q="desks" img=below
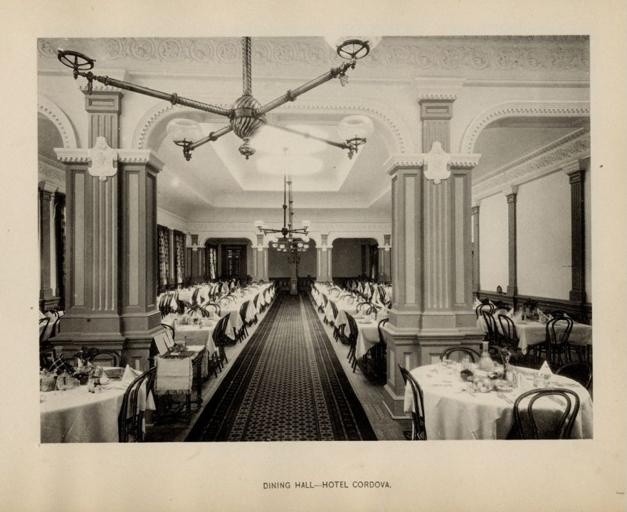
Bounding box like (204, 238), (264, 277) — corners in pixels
(407, 362), (593, 439)
(482, 307), (592, 367)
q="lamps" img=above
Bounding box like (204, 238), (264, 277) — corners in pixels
(255, 220), (310, 252)
(57, 20), (371, 160)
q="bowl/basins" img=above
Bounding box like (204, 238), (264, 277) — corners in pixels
(72, 373), (89, 386)
(106, 368), (126, 380)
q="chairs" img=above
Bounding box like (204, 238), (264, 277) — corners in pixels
(514, 387), (580, 438)
(475, 303), (526, 362)
(397, 362), (427, 440)
(309, 274), (395, 382)
(40, 313), (157, 442)
(157, 277), (275, 368)
(555, 361), (592, 389)
(440, 346), (484, 363)
(532, 316), (574, 369)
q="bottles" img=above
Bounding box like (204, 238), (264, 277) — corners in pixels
(41, 370), (74, 393)
(478, 340), (495, 371)
